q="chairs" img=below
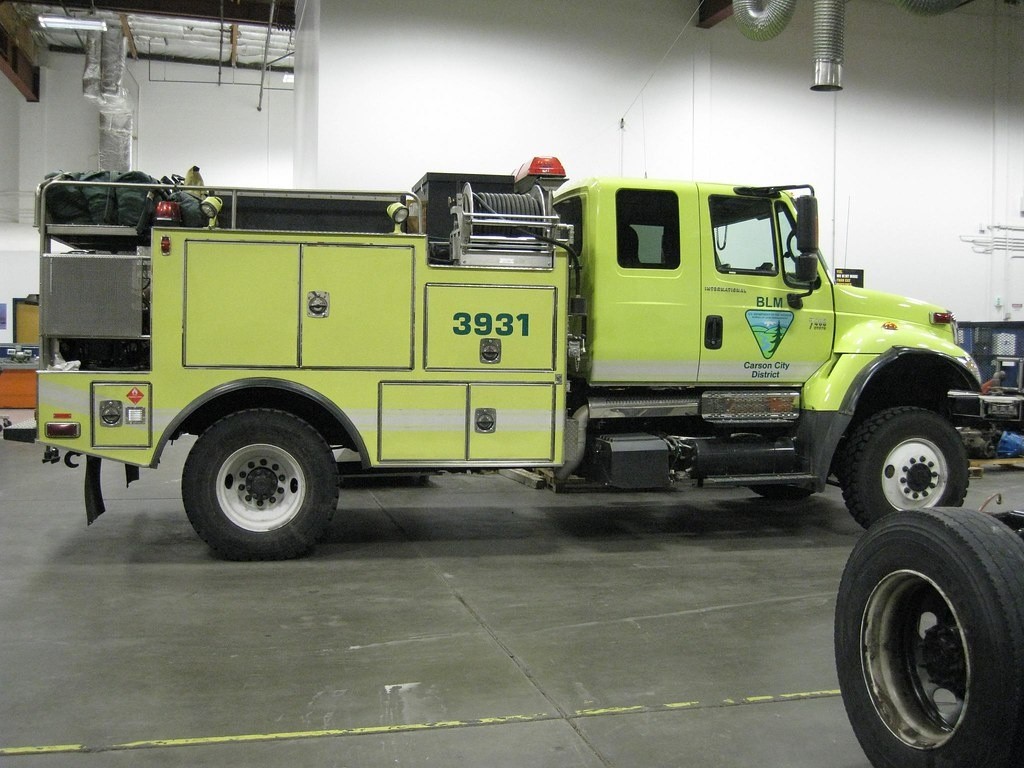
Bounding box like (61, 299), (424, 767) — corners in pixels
(660, 230), (679, 260)
(620, 222), (643, 264)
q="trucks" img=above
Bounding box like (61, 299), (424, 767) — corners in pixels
(34, 158), (991, 559)
